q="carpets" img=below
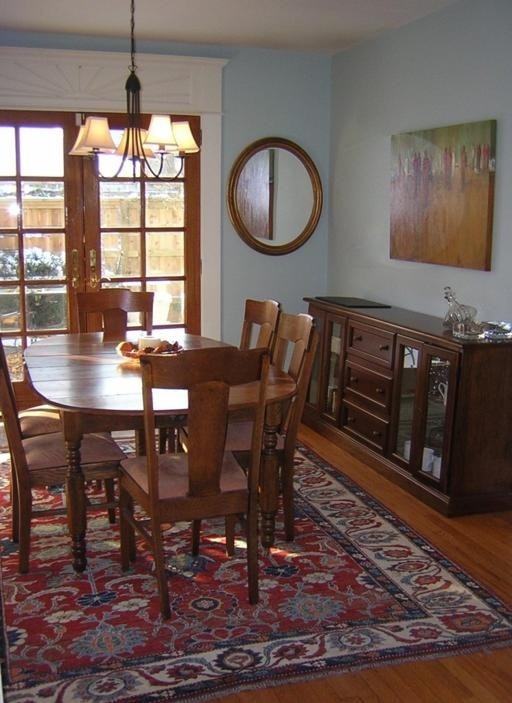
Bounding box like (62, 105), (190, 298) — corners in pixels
(0, 416), (512, 703)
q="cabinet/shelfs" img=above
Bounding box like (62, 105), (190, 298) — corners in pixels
(300, 302), (512, 517)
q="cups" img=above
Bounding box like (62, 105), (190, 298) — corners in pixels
(422, 447), (442, 478)
(404, 440), (412, 461)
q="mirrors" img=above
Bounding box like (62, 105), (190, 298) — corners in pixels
(226, 136), (324, 255)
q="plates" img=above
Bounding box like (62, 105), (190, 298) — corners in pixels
(115, 345), (183, 359)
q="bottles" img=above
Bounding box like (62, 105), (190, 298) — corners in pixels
(442, 286), (478, 327)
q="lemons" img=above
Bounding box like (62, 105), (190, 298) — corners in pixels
(118, 341), (132, 354)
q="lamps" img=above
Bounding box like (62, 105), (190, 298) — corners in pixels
(69, 0), (201, 182)
